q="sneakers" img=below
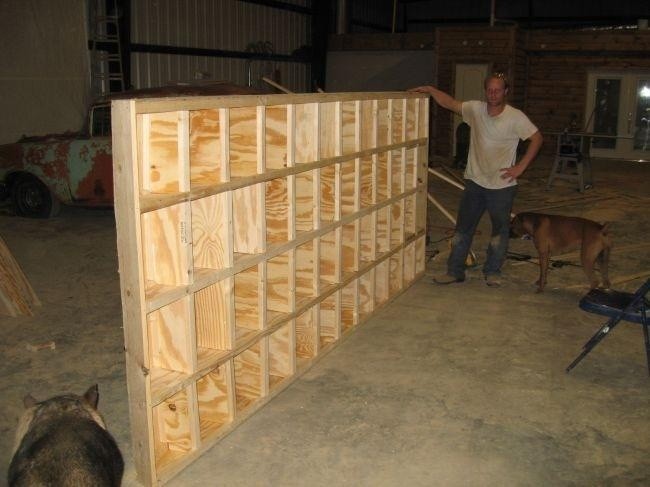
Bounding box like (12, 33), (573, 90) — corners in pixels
(485, 274), (502, 286)
(433, 274), (457, 284)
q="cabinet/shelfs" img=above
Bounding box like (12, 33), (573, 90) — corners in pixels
(110, 90), (429, 487)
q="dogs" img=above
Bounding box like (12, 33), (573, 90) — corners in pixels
(8, 381), (126, 487)
(506, 210), (615, 294)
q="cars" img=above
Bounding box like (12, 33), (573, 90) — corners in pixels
(1, 80), (274, 220)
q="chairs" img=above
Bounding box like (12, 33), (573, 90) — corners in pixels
(564, 277), (650, 372)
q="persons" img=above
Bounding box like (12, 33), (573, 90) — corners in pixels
(407, 71), (546, 286)
